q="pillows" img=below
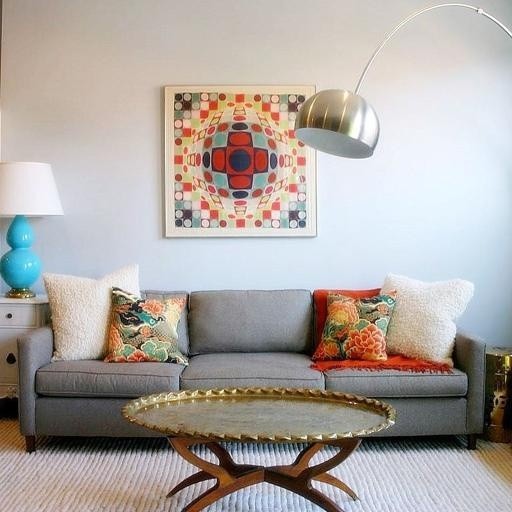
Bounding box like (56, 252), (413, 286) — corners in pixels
(314, 288), (380, 349)
(312, 292), (396, 362)
(42, 265), (141, 362)
(104, 287), (190, 367)
(379, 275), (475, 368)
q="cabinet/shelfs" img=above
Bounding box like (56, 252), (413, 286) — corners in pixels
(0, 294), (51, 422)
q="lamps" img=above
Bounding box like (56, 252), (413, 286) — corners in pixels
(294, 3), (512, 159)
(0, 163), (63, 298)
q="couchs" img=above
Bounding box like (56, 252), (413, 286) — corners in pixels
(15, 290), (486, 454)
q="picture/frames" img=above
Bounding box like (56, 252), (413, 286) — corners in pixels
(164, 85), (317, 238)
